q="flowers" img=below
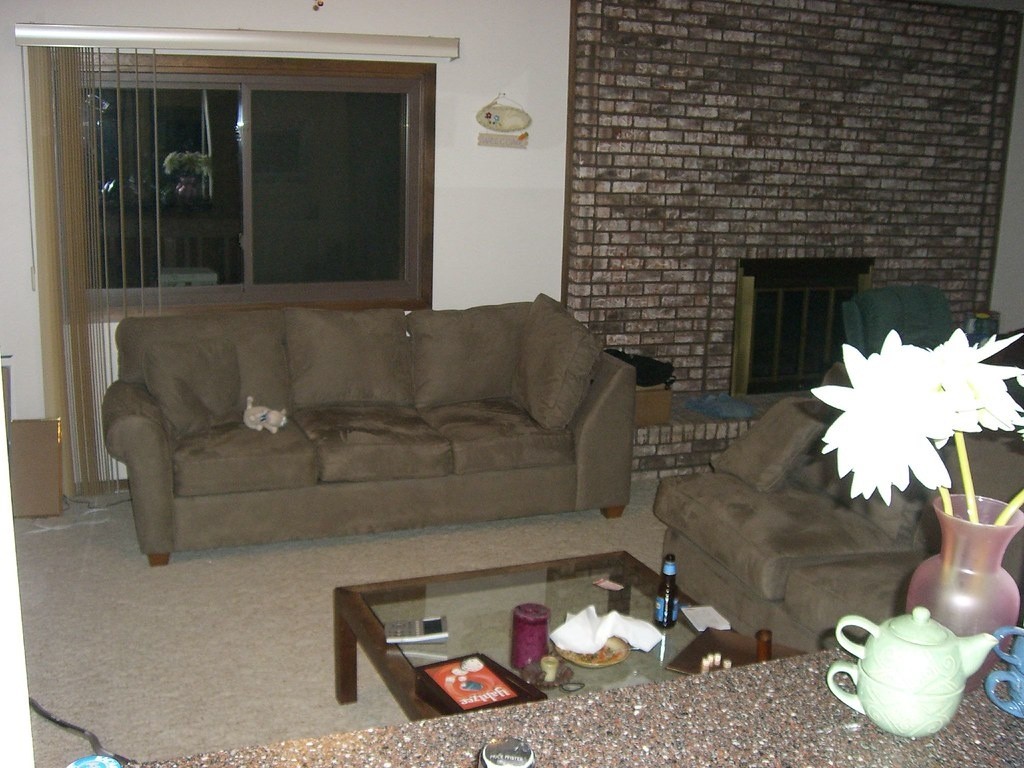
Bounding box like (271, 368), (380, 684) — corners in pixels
(165, 153), (212, 181)
(812, 325), (1024, 519)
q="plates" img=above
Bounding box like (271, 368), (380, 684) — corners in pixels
(554, 636), (631, 668)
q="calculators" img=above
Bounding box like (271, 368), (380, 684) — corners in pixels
(384, 614), (450, 644)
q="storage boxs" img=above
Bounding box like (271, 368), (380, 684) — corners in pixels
(630, 368), (675, 428)
(9, 419), (63, 518)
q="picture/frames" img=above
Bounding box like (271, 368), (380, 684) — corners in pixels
(414, 653), (548, 717)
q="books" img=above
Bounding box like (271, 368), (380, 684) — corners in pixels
(665, 627), (811, 676)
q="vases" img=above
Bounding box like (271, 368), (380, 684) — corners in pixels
(174, 176), (200, 207)
(893, 496), (1018, 695)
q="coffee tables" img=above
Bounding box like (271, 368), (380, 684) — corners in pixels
(332, 547), (750, 722)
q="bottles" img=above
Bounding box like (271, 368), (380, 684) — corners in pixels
(654, 554), (679, 629)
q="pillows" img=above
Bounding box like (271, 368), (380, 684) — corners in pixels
(509, 294), (606, 431)
(142, 335), (243, 445)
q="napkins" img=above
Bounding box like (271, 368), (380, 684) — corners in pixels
(551, 603), (662, 653)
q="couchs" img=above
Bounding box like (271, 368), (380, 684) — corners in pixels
(653, 353), (1014, 651)
(842, 287), (959, 351)
(102, 295), (629, 565)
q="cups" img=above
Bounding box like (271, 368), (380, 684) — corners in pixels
(985, 621), (1024, 719)
(827, 661), (964, 737)
(540, 656), (559, 682)
(511, 604), (551, 668)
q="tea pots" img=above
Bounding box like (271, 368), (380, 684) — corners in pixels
(836, 607), (999, 695)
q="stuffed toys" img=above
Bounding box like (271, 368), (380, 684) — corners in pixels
(243, 397), (287, 434)
